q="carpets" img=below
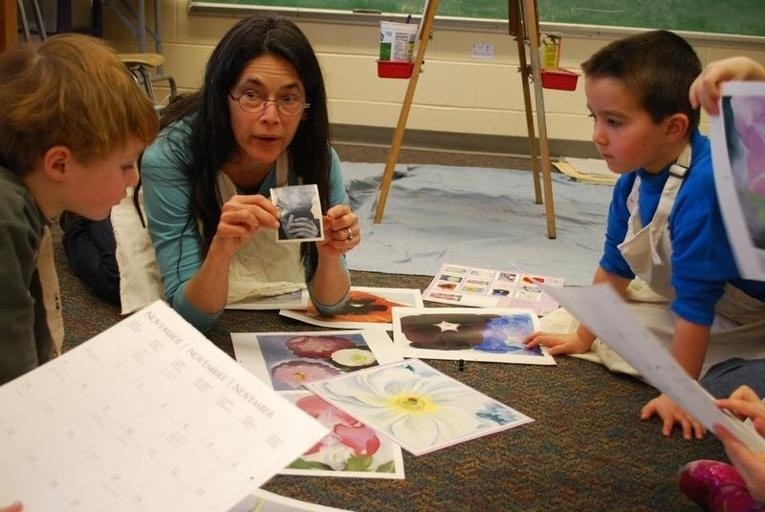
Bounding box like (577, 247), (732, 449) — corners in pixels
(2, 123), (733, 511)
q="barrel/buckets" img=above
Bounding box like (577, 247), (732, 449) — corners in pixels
(378, 22), (418, 62)
(538, 30), (562, 71)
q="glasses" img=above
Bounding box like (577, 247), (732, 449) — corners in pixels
(228, 87), (313, 117)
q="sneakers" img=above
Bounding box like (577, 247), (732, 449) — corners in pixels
(677, 454), (765, 511)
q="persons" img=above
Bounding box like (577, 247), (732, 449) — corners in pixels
(58, 15), (363, 335)
(681, 384), (764, 511)
(1, 32), (159, 389)
(523, 28), (764, 442)
(273, 185), (321, 240)
(688, 56), (765, 404)
(0, 501), (24, 511)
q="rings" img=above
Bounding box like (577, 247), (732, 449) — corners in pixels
(347, 227), (353, 240)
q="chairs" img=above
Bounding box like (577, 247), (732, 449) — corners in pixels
(18, 0), (175, 228)
